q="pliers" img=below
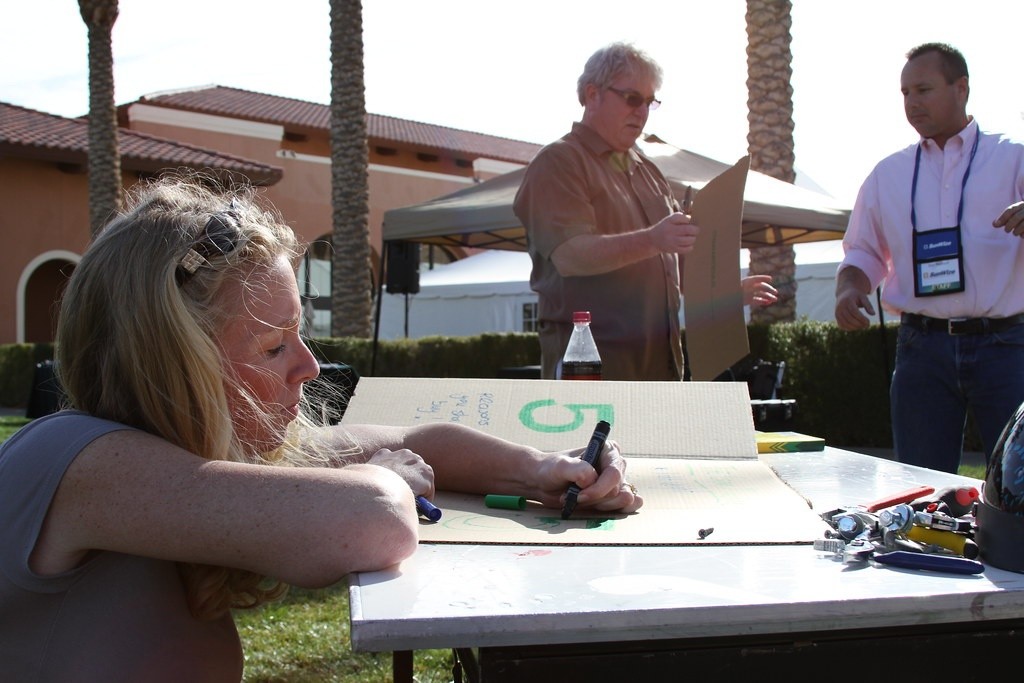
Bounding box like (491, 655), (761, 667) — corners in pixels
(818, 485), (979, 559)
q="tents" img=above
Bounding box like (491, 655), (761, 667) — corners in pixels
(368, 134), (884, 378)
(378, 249), (538, 340)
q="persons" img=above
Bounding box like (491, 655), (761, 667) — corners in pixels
(0, 182), (644, 683)
(835, 44), (1023, 477)
(513, 43), (778, 385)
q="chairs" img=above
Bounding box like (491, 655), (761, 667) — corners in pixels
(751, 357), (786, 426)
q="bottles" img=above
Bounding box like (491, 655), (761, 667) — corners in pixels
(560, 311), (603, 380)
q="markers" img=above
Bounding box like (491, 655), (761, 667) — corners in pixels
(561, 420), (611, 520)
(416, 495), (442, 522)
(873, 551), (984, 576)
(684, 186), (692, 214)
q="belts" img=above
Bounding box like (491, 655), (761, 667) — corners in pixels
(901, 312), (1023, 336)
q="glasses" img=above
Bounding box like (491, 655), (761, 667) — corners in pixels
(603, 84), (661, 110)
(176, 199), (244, 289)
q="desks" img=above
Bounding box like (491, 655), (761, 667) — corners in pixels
(348, 429), (1024, 683)
(751, 398), (798, 409)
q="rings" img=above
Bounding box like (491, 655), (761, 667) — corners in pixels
(620, 483), (637, 495)
